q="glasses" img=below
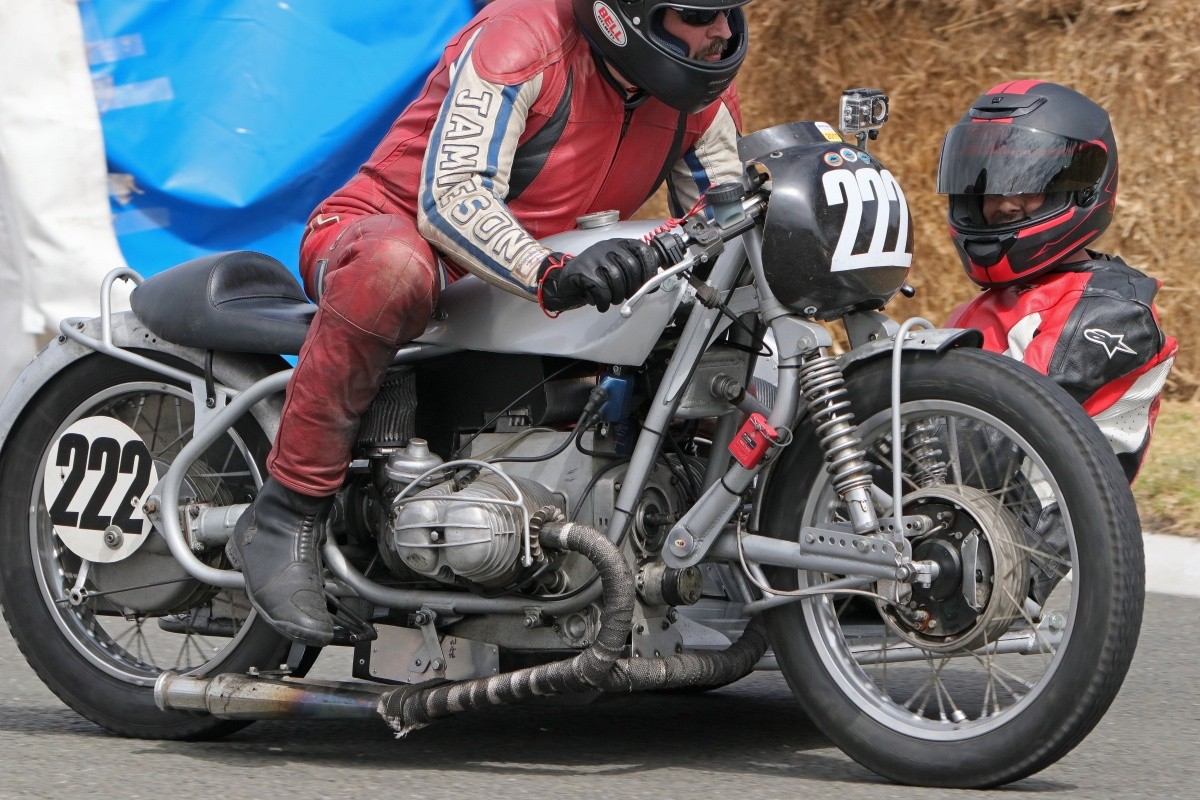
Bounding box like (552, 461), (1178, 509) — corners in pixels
(669, 6), (731, 26)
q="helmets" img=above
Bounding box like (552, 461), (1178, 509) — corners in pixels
(571, 0), (752, 114)
(936, 79), (1118, 287)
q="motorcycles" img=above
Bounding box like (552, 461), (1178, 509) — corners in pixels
(1, 85), (1147, 793)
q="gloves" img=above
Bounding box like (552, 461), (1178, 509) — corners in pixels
(537, 237), (660, 313)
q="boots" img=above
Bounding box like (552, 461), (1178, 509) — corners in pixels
(223, 474), (336, 647)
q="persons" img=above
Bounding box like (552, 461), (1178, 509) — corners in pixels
(224, 0), (746, 646)
